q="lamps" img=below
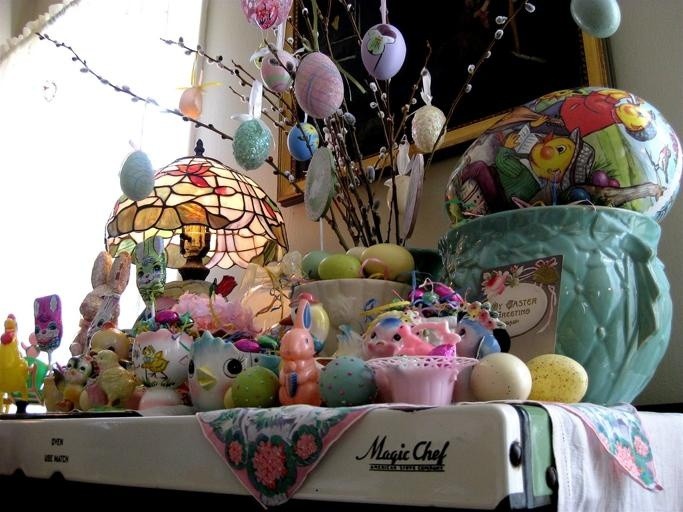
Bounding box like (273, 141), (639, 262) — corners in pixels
(99, 136), (291, 339)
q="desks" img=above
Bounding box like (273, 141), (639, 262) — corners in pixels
(0, 406), (683, 511)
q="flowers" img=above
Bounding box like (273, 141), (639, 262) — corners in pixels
(30, 2), (542, 254)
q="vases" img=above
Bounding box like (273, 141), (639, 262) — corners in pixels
(281, 270), (414, 363)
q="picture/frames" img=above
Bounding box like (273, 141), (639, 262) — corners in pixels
(274, 1), (618, 204)
